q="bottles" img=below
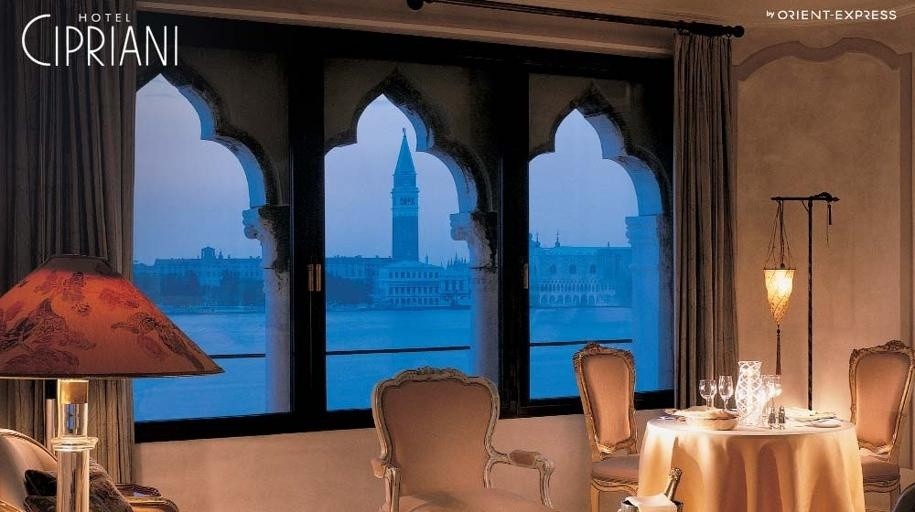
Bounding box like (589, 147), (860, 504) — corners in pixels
(735, 361), (767, 426)
(768, 406), (785, 423)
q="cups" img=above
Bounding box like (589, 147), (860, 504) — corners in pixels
(762, 375), (782, 413)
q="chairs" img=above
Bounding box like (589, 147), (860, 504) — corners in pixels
(847, 336), (913, 511)
(571, 340), (644, 511)
(370, 367), (556, 512)
(1, 426), (180, 512)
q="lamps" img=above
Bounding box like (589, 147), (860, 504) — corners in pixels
(0, 256), (226, 512)
(763, 201), (795, 326)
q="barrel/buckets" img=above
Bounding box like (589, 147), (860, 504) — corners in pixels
(621, 500), (684, 512)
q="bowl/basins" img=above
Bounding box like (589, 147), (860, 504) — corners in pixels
(683, 415), (740, 430)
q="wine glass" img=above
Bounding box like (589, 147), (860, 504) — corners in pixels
(698, 376), (733, 412)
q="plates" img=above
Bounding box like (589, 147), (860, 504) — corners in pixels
(812, 419), (842, 428)
